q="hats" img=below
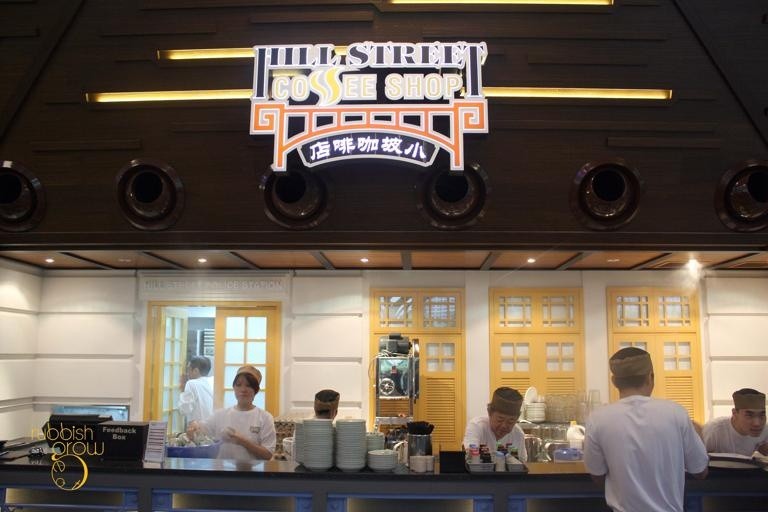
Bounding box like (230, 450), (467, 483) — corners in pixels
(236, 367), (262, 384)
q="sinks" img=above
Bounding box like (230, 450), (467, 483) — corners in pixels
(521, 427), (566, 443)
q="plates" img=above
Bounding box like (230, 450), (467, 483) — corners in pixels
(514, 388), (546, 424)
(291, 416), (399, 474)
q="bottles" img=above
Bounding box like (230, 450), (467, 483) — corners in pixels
(467, 439), (519, 472)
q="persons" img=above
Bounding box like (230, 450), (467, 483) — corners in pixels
(186, 366), (276, 463)
(582, 348), (710, 512)
(179, 355), (214, 431)
(314, 390), (340, 421)
(464, 388), (528, 463)
(703, 388), (768, 457)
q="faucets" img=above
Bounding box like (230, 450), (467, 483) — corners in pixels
(394, 441), (408, 463)
(516, 420), (531, 425)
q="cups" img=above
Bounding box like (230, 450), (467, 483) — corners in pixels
(524, 436), (542, 462)
(544, 389), (600, 424)
(410, 454), (436, 473)
(404, 433), (433, 465)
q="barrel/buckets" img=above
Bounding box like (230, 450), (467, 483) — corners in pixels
(566, 421), (585, 450)
